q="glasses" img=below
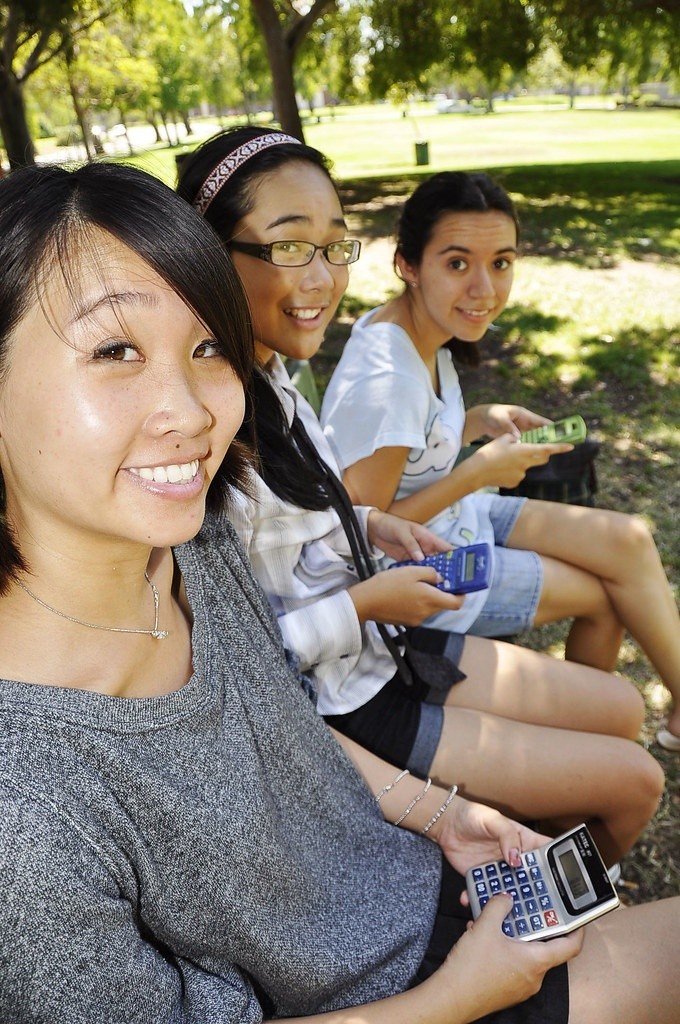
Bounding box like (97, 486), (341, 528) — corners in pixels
(224, 239), (361, 265)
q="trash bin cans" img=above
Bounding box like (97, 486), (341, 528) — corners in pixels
(414, 140), (429, 165)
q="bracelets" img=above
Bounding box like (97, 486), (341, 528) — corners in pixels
(374, 769), (458, 832)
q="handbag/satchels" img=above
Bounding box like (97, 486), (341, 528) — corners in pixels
(495, 441), (613, 516)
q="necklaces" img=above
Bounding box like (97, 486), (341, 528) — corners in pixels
(12, 570), (169, 642)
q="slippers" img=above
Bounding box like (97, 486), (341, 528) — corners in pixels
(657, 728), (679, 751)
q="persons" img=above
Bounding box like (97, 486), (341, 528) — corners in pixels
(320, 169), (680, 751)
(166, 126), (664, 885)
(2, 160), (680, 1024)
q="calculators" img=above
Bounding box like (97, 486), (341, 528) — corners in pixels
(385, 542), (493, 596)
(516, 414), (587, 447)
(463, 821), (621, 942)
